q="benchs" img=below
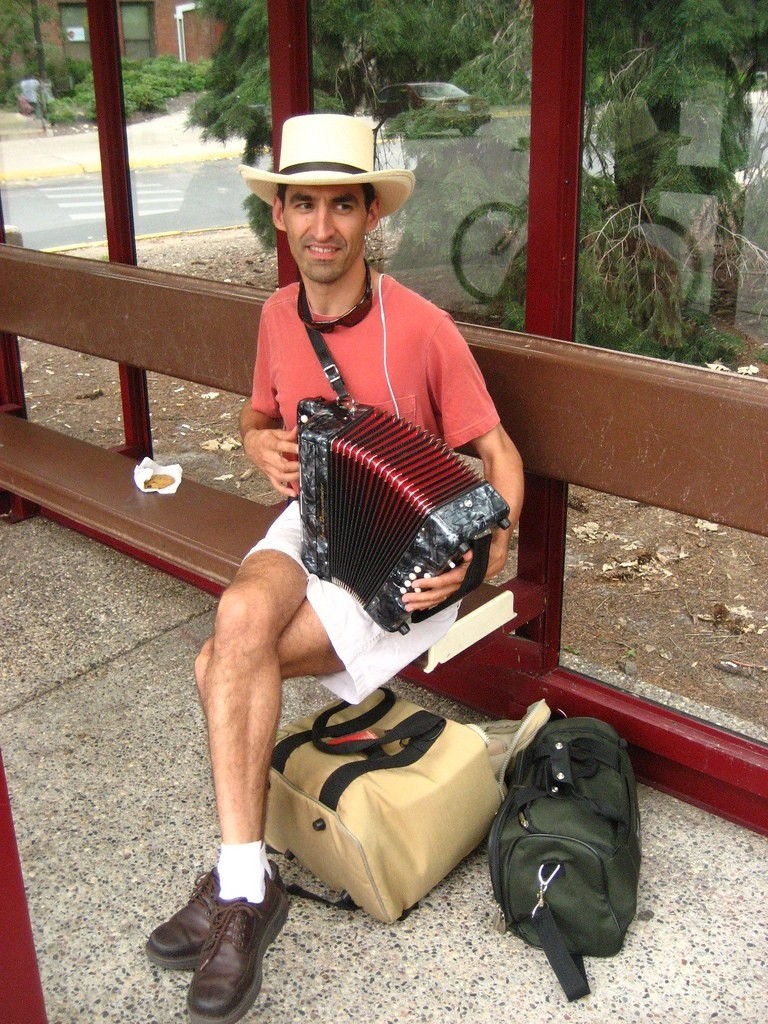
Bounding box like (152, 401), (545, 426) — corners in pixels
(0, 411), (519, 676)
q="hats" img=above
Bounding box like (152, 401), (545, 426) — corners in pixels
(239, 114), (416, 217)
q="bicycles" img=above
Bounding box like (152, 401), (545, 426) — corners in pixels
(450, 142), (704, 304)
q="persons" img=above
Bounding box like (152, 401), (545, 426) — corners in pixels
(146, 111), (526, 1024)
(20, 70), (55, 128)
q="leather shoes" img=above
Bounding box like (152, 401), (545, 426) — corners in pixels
(145, 858), (290, 970)
(184, 869), (288, 1024)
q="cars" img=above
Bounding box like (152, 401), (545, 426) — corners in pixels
(370, 82), (492, 139)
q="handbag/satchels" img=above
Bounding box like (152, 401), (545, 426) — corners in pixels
(258, 686), (551, 927)
(486, 714), (642, 1003)
(15, 94), (35, 115)
